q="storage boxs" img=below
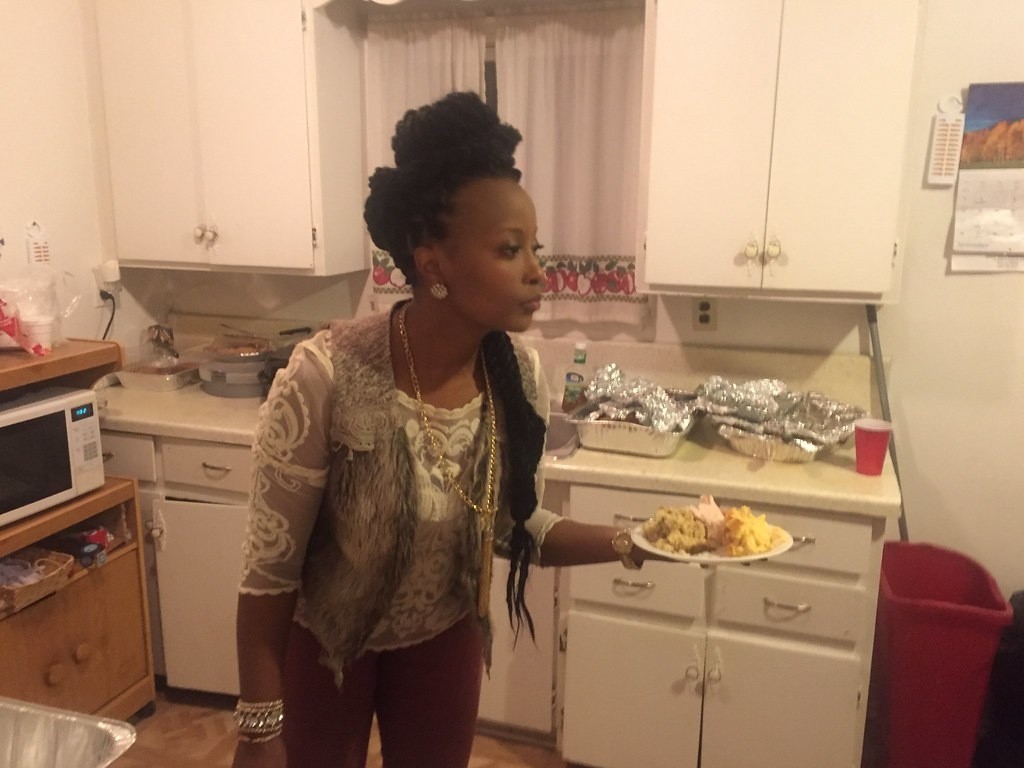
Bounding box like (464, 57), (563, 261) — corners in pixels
(562, 388), (702, 457)
(718, 423), (824, 464)
(114, 360), (200, 391)
(0, 696), (138, 768)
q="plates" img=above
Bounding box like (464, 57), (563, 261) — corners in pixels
(629, 505), (794, 564)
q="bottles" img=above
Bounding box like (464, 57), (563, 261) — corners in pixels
(560, 342), (588, 414)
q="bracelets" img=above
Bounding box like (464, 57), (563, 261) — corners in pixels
(233, 701), (285, 743)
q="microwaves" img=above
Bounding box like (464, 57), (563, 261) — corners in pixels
(0, 389), (106, 529)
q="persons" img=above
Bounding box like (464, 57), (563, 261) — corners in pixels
(231, 86), (773, 768)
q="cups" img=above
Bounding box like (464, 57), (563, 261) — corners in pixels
(20, 315), (56, 353)
(854, 418), (894, 478)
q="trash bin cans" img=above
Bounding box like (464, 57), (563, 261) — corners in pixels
(861, 541), (1014, 768)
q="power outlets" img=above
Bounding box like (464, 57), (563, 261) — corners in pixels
(692, 297), (717, 332)
(90, 269), (108, 308)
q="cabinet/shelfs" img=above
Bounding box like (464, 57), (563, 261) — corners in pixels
(97, 0), (372, 281)
(93, 312), (322, 697)
(633, 0), (929, 306)
(540, 341), (902, 768)
(0, 338), (158, 718)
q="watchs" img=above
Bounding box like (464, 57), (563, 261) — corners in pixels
(613, 524), (643, 572)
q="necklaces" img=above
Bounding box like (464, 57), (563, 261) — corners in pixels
(398, 299), (497, 618)
